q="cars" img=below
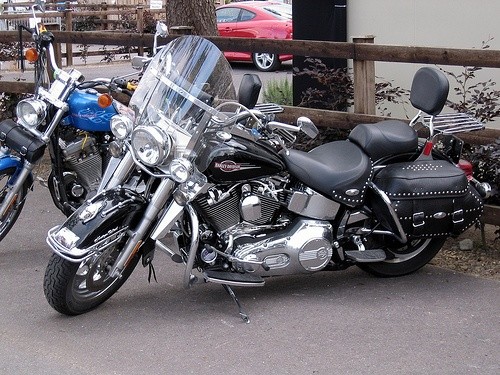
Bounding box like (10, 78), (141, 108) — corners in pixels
(215, 1), (293, 72)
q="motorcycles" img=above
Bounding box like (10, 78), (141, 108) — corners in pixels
(0, 2), (493, 325)
